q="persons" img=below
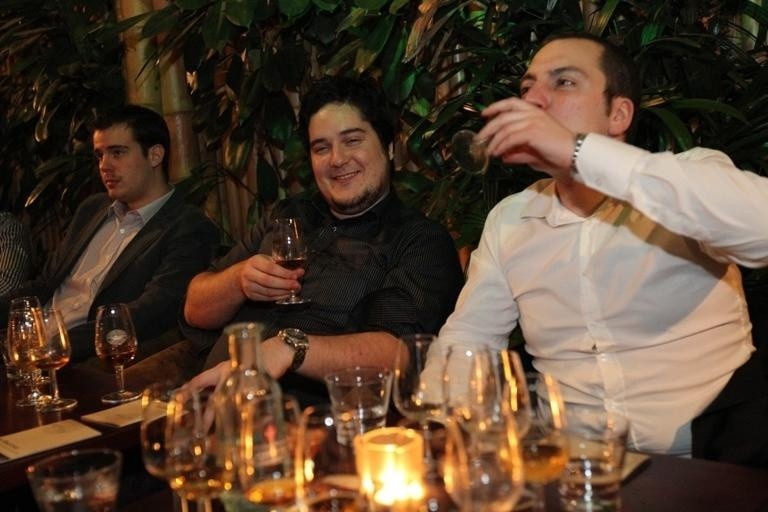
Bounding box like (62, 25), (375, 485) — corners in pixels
(0, 210), (32, 328)
(170, 77), (462, 435)
(415, 37), (768, 460)
(0, 103), (221, 374)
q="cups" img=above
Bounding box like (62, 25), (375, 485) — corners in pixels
(25, 447), (125, 512)
(239, 393), (303, 507)
(351, 426), (425, 512)
(321, 364), (393, 448)
(298, 400), (369, 511)
(556, 410), (632, 512)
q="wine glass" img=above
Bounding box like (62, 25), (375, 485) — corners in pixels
(393, 328), (569, 512)
(138, 382), (234, 512)
(0, 295), (80, 415)
(94, 302), (143, 405)
(270, 216), (312, 305)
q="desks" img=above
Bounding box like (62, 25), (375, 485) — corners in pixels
(0, 386), (768, 511)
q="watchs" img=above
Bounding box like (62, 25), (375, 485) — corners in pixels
(277, 328), (310, 374)
(570, 132), (585, 174)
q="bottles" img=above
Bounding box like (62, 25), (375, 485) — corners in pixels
(212, 320), (298, 509)
